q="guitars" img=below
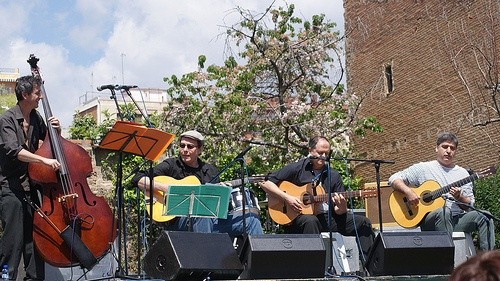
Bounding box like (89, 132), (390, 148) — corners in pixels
(145, 173), (266, 222)
(388, 165), (496, 229)
(268, 180), (377, 225)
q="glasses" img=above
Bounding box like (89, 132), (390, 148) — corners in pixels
(178, 143), (200, 148)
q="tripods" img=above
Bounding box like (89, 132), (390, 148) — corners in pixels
(87, 130), (159, 280)
(315, 149), (346, 278)
(124, 158), (157, 281)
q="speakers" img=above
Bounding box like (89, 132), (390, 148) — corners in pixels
(235, 234), (326, 278)
(365, 233), (455, 276)
(141, 231), (244, 281)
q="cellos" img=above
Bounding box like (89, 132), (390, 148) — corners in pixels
(27, 54), (117, 269)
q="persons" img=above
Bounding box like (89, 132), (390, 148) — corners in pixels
(387, 133), (496, 256)
(131, 129), (265, 243)
(450, 246), (500, 281)
(0, 75), (63, 281)
(260, 135), (377, 276)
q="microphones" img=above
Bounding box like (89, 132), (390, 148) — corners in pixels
(114, 84), (138, 92)
(442, 193), (458, 202)
(97, 83), (116, 92)
(311, 180), (318, 196)
(307, 153), (319, 160)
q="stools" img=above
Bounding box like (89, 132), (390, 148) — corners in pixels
(319, 231), (357, 275)
(373, 225), (477, 270)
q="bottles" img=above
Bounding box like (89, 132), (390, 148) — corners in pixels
(2, 264), (9, 281)
